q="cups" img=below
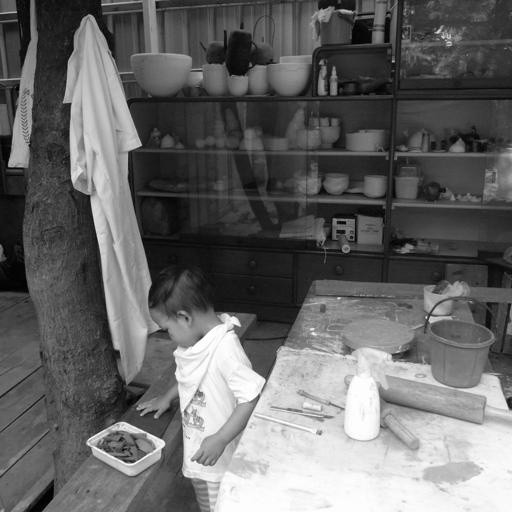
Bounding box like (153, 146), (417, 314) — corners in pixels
(237, 126), (289, 151)
(201, 63), (228, 96)
(423, 283), (454, 315)
(343, 82), (358, 95)
(246, 64), (270, 95)
(226, 75), (249, 97)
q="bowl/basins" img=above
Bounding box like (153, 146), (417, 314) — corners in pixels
(268, 62), (312, 98)
(181, 67), (205, 98)
(309, 126), (341, 149)
(129, 52), (193, 98)
(295, 129), (321, 151)
(291, 168), (389, 199)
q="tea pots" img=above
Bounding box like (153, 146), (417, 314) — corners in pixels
(222, 21), (259, 76)
(249, 14), (277, 66)
(198, 40), (227, 65)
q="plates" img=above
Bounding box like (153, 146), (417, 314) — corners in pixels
(344, 128), (389, 152)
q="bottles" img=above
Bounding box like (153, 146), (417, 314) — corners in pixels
(329, 65), (339, 97)
(421, 133), (431, 153)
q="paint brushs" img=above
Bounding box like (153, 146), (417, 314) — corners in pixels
(270, 406), (334, 422)
(254, 413), (323, 435)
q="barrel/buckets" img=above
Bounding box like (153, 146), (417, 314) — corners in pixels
(424, 295), (500, 390)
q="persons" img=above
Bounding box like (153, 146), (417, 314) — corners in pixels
(135, 262), (267, 511)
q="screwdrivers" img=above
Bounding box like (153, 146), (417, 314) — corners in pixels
(297, 388), (346, 411)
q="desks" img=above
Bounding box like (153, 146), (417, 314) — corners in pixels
(215, 279), (511, 511)
